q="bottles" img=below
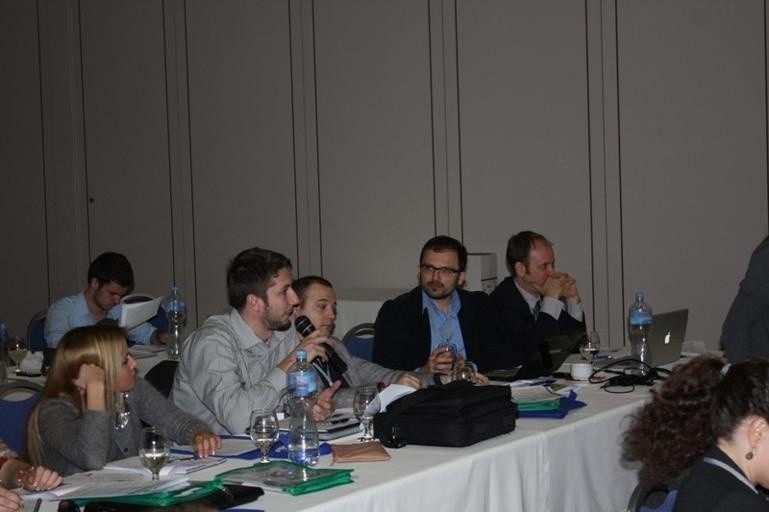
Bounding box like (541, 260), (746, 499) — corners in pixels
(287, 389), (320, 466)
(628, 292), (653, 374)
(166, 286), (186, 359)
(286, 349), (322, 452)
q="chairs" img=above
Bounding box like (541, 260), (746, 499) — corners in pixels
(1, 381), (44, 455)
(120, 294), (169, 333)
(140, 360), (178, 449)
(28, 310), (47, 349)
(341, 322), (376, 362)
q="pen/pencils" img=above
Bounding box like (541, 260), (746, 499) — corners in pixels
(167, 457), (196, 463)
(34, 498), (41, 511)
(21, 505), (26, 508)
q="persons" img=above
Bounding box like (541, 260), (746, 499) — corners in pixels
(722, 236), (769, 365)
(43, 252), (172, 349)
(372, 236), (506, 376)
(0, 440), (63, 512)
(621, 358), (769, 512)
(289, 276), (491, 409)
(167, 248), (342, 435)
(24, 326), (222, 477)
(489, 231), (589, 382)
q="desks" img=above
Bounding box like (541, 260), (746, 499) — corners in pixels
(1, 340), (707, 512)
(0, 346), (178, 402)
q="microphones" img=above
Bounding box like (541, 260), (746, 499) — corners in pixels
(294, 313), (347, 374)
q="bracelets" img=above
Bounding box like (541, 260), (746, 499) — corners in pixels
(17, 465), (34, 489)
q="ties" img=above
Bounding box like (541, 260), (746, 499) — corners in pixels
(533, 301), (540, 322)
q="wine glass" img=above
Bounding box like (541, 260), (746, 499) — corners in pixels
(437, 343), (457, 384)
(139, 427), (170, 482)
(354, 388), (381, 442)
(250, 409), (279, 464)
(8, 336), (28, 373)
(579, 331), (600, 363)
(451, 362), (478, 384)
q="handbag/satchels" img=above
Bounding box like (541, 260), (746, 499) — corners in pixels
(57, 480), (264, 512)
(373, 379), (518, 448)
(214, 460), (354, 495)
(516, 398), (560, 410)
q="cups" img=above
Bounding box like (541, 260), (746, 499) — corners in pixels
(570, 363), (593, 380)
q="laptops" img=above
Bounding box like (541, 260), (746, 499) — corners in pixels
(592, 309), (689, 374)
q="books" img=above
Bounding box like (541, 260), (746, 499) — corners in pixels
(279, 417), (364, 441)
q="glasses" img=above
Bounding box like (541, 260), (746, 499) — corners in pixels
(420, 264), (459, 275)
(15, 468), (34, 512)
